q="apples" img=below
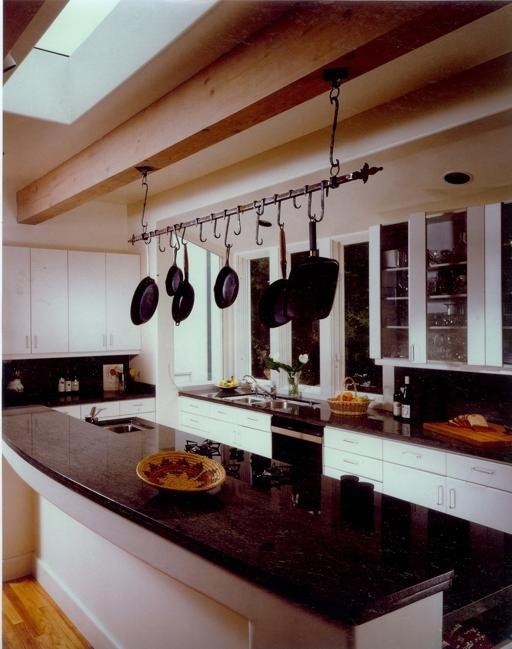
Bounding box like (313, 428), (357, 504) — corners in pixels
(337, 392), (364, 403)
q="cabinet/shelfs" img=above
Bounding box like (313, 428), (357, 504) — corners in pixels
(177, 203), (510, 416)
(3, 246), (156, 422)
(381, 442), (511, 532)
(321, 427), (383, 494)
(179, 396), (271, 457)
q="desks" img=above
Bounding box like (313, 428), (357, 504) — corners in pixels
(3, 406), (454, 649)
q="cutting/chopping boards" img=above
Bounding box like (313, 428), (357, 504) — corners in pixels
(423, 420), (512, 447)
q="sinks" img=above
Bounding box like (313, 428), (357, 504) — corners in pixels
(227, 396), (270, 402)
(98, 419), (154, 433)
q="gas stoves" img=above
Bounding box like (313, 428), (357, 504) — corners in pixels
(181, 439), (280, 490)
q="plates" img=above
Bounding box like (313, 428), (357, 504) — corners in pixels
(217, 387), (239, 391)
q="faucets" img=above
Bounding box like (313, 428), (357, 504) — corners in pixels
(242, 374), (276, 400)
(89, 407), (108, 424)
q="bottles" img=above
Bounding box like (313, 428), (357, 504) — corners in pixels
(59, 376), (80, 392)
(393, 375), (413, 423)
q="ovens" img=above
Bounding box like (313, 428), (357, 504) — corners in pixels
(271, 416), (323, 475)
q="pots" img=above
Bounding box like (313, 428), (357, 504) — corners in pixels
(130, 218), (339, 327)
